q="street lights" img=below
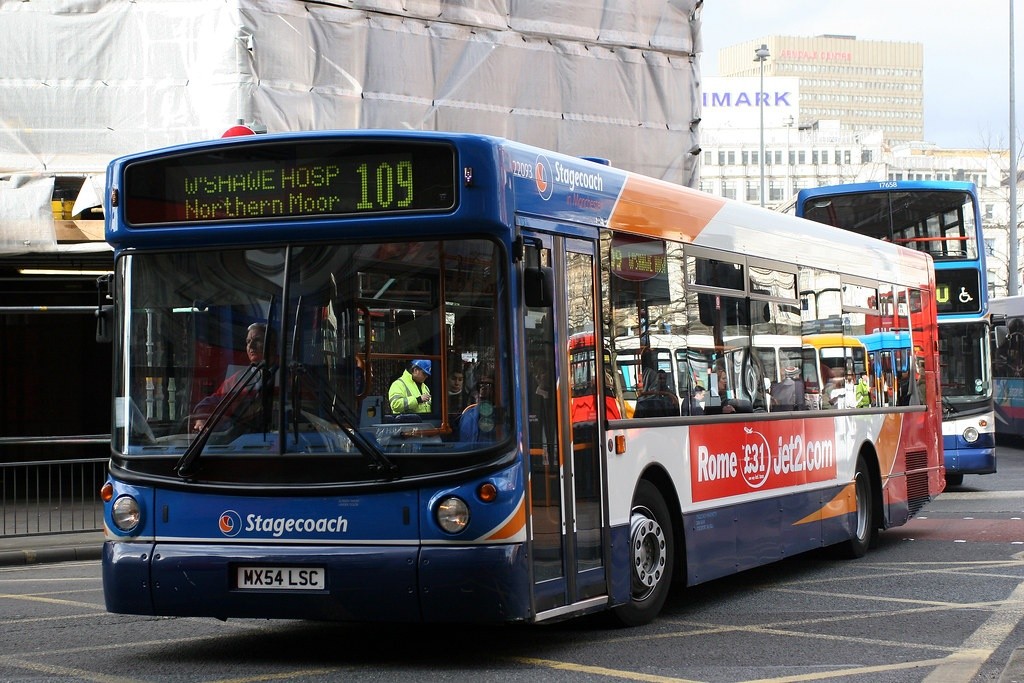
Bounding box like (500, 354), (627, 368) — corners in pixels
(753, 44), (770, 207)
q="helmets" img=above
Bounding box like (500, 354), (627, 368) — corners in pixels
(412, 360), (432, 375)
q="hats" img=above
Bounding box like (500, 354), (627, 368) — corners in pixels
(786, 366), (802, 377)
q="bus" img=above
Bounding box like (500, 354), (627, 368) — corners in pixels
(94, 126), (946, 623)
(795, 181), (996, 486)
(988, 296), (1024, 438)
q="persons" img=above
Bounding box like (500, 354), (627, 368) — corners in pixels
(192, 321), (282, 430)
(603, 346), (927, 419)
(388, 359), (431, 415)
(449, 360), (557, 503)
